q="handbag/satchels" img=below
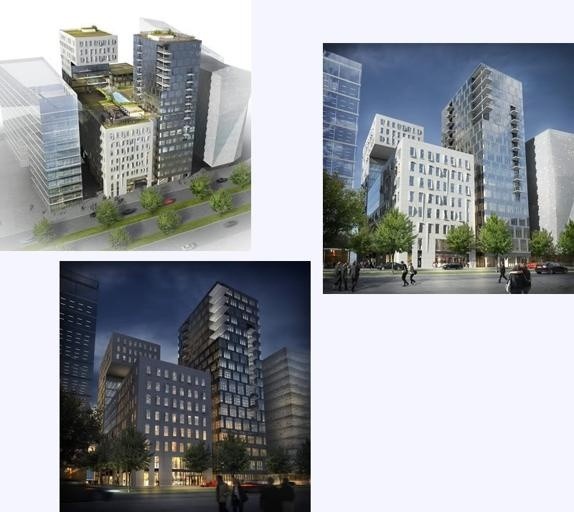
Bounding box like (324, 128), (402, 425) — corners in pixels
(414, 270), (417, 275)
(506, 280), (512, 293)
